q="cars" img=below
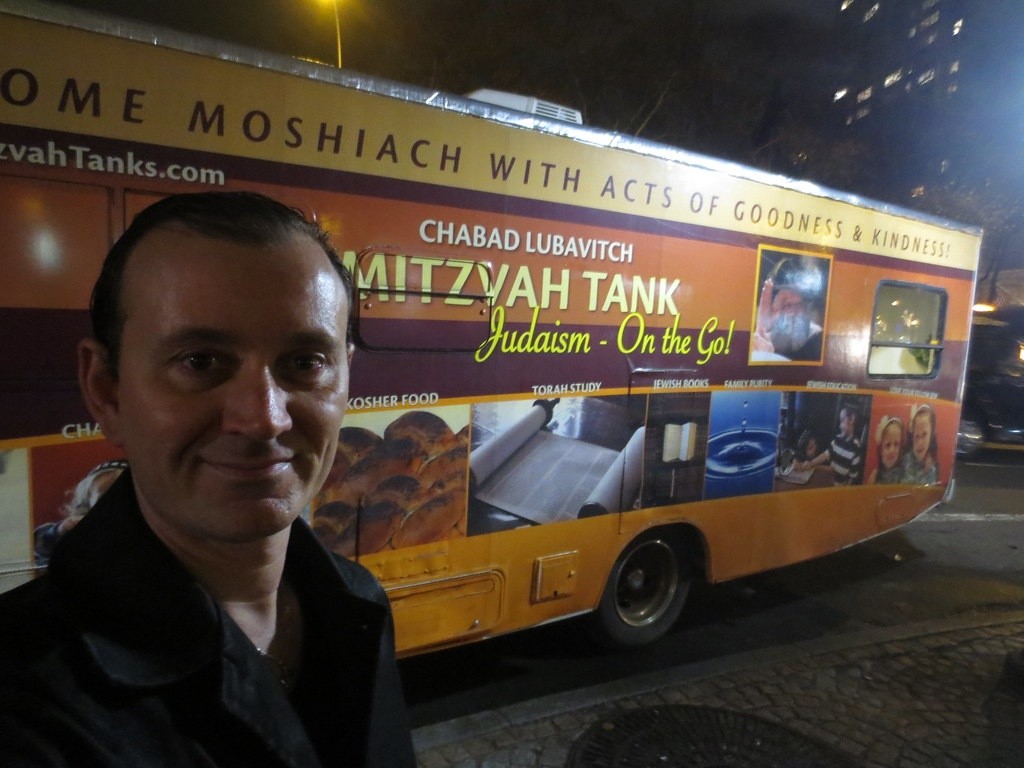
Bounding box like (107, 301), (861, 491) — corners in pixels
(959, 301), (1024, 442)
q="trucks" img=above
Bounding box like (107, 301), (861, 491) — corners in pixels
(0, 4), (989, 667)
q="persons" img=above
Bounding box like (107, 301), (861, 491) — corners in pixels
(0, 192), (417, 768)
(798, 430), (819, 464)
(791, 402), (867, 486)
(899, 404), (938, 484)
(867, 415), (910, 484)
(31, 460), (128, 559)
(752, 260), (822, 362)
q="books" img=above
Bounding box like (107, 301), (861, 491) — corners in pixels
(663, 422), (697, 462)
